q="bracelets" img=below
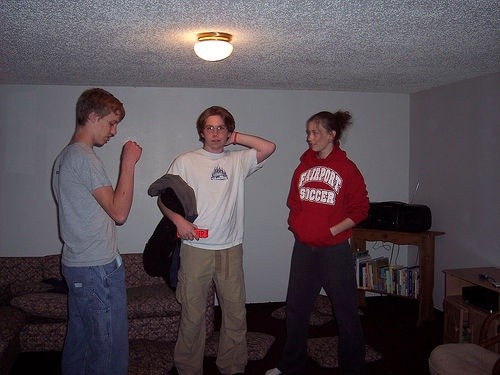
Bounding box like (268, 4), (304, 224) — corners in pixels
(234, 132), (239, 144)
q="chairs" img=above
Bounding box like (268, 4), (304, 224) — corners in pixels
(428, 309), (500, 375)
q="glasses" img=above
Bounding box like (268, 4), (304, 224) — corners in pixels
(204, 125), (228, 133)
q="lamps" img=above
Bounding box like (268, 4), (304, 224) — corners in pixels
(193, 31), (234, 61)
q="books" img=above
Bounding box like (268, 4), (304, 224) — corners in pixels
(354, 252), (421, 299)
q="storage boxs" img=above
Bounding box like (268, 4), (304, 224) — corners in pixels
(176, 229), (208, 238)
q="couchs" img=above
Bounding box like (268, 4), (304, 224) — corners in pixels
(1, 253), (214, 356)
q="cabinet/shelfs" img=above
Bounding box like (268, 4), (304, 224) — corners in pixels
(353, 226), (446, 329)
(441, 266), (500, 350)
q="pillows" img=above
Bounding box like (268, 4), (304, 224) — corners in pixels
(202, 329), (273, 361)
(126, 337), (177, 375)
(309, 337), (382, 368)
(273, 293), (334, 326)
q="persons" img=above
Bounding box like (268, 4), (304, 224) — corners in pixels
(50, 88), (142, 374)
(157, 106), (276, 375)
(265, 111), (369, 375)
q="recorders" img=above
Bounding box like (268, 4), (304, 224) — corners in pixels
(356, 182), (432, 233)
